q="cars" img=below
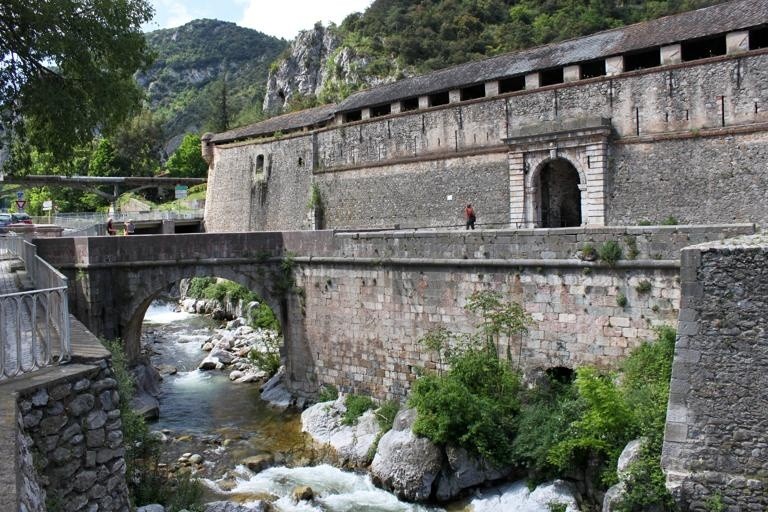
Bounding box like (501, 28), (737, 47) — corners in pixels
(0, 212), (32, 232)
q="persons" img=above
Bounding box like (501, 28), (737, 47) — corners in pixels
(127, 219), (135, 234)
(107, 218), (113, 235)
(465, 202), (475, 230)
